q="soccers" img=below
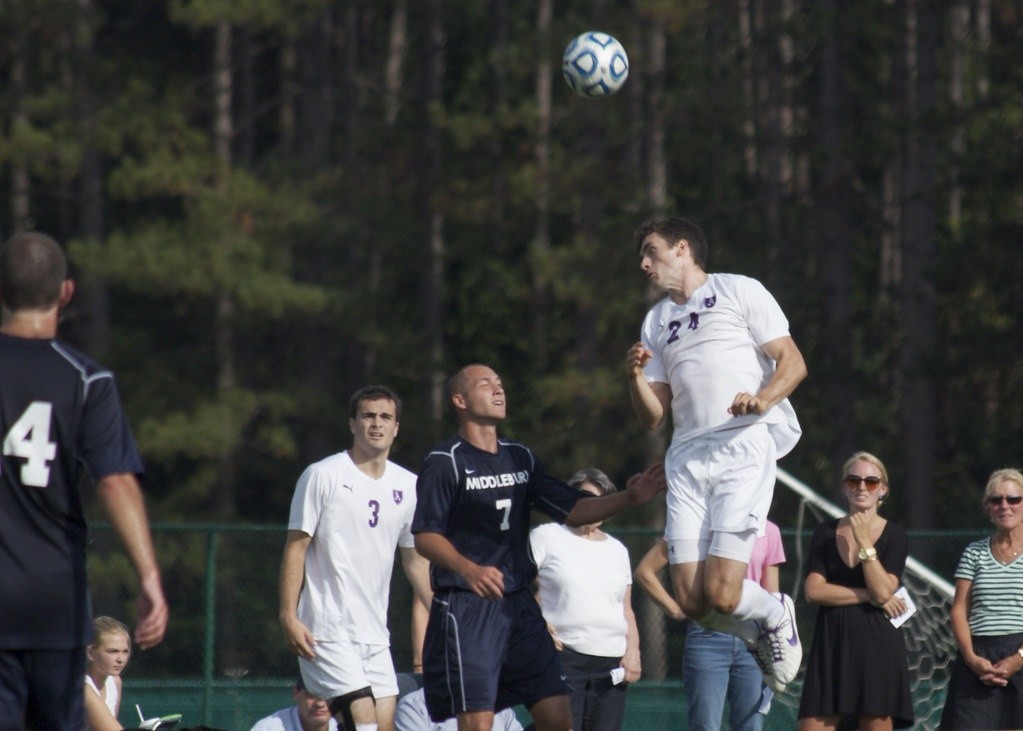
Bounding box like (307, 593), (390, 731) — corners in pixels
(562, 31), (630, 99)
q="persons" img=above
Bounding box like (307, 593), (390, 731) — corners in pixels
(410, 364), (668, 731)
(631, 519), (786, 731)
(249, 677), (338, 731)
(529, 469), (642, 731)
(0, 232), (169, 731)
(939, 468), (1023, 731)
(84, 616), (131, 731)
(796, 451), (915, 731)
(624, 216), (808, 693)
(394, 687), (524, 731)
(278, 385), (434, 731)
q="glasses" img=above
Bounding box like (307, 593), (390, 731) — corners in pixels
(988, 496), (1023, 505)
(843, 475), (882, 491)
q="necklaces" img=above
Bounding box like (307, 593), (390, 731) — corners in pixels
(994, 535), (1023, 565)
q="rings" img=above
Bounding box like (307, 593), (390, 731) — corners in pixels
(892, 607), (896, 610)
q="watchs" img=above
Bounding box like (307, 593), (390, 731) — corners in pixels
(858, 548), (876, 561)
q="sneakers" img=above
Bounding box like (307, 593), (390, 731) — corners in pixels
(747, 620), (786, 692)
(760, 592), (802, 683)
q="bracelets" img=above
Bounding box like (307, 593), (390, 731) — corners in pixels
(413, 664), (422, 667)
(1017, 648), (1023, 657)
